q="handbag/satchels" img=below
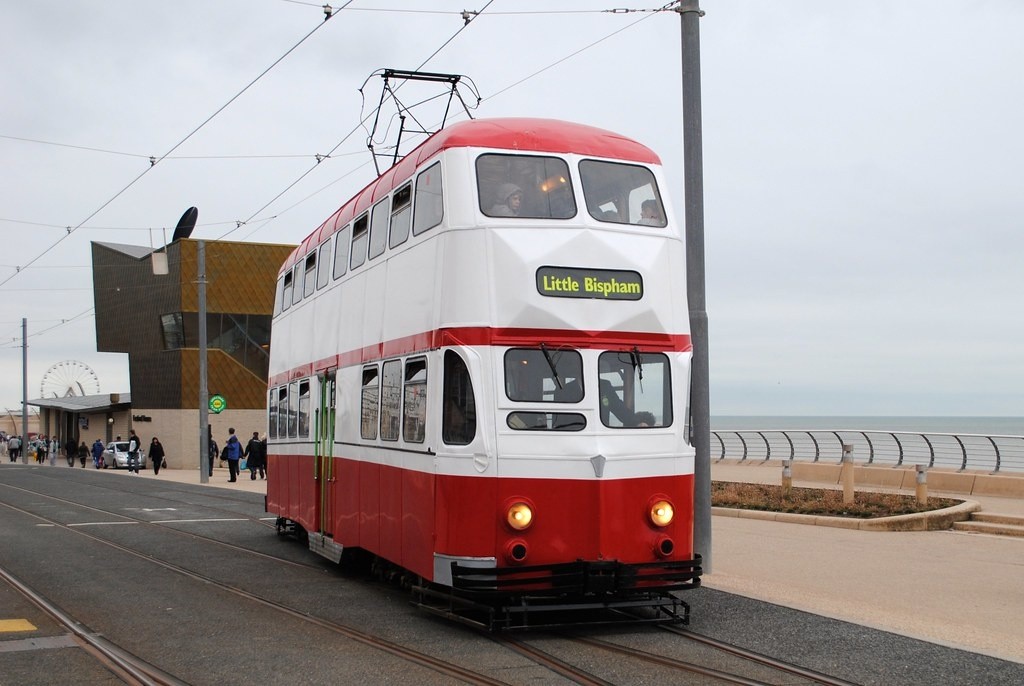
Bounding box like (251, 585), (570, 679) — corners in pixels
(162, 459), (167, 469)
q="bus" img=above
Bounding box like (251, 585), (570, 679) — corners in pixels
(266, 117), (704, 635)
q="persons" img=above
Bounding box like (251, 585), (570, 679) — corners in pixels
(211, 428), (267, 482)
(556, 358), (656, 432)
(148, 437), (164, 475)
(0, 433), (122, 469)
(128, 430), (141, 475)
(479, 167), (667, 227)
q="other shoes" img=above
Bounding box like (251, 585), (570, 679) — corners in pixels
(155, 470), (158, 475)
(81, 464), (86, 468)
(209, 471), (212, 476)
(227, 479), (236, 482)
(129, 469), (139, 475)
(251, 471), (256, 480)
(260, 472), (265, 478)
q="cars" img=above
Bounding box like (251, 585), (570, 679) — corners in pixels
(100, 441), (146, 470)
